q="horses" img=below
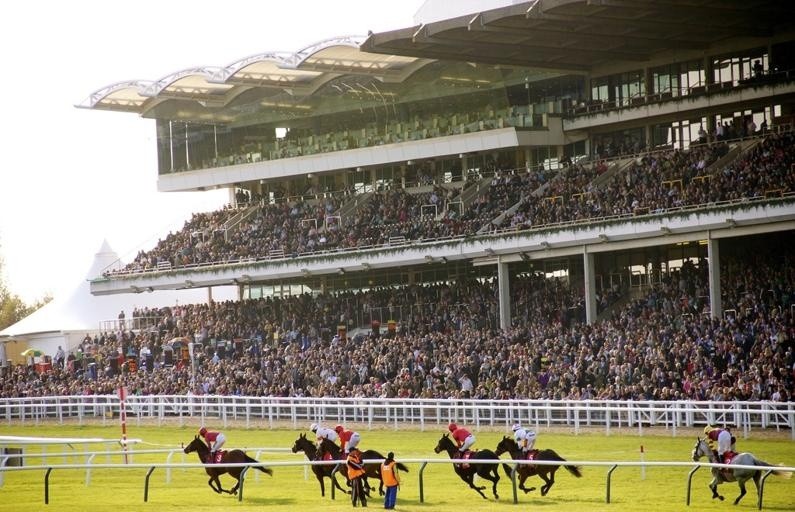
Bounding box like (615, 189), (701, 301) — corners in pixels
(691, 435), (793, 508)
(495, 434), (581, 496)
(433, 432), (513, 500)
(291, 431), (409, 499)
(183, 434), (273, 496)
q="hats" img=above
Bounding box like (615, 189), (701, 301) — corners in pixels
(349, 447), (357, 452)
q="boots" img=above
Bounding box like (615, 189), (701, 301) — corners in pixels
(718, 455), (724, 470)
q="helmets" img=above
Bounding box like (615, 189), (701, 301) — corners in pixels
(310, 424), (318, 430)
(449, 423), (456, 430)
(335, 426), (343, 432)
(199, 427), (206, 435)
(512, 424), (521, 431)
(704, 425), (713, 435)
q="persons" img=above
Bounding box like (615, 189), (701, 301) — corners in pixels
(346, 447), (368, 508)
(511, 424), (537, 469)
(198, 427), (225, 462)
(702, 425), (731, 471)
(380, 452), (400, 511)
(333, 425), (360, 460)
(724, 427), (736, 453)
(448, 424), (475, 468)
(310, 423), (337, 461)
